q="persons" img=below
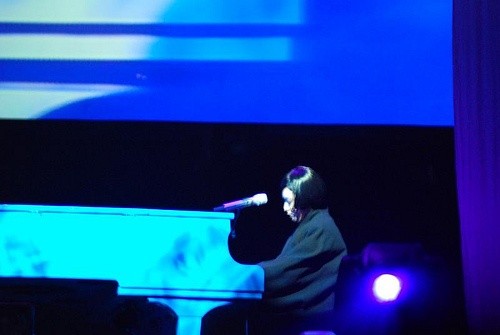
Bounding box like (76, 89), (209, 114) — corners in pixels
(199, 165), (350, 335)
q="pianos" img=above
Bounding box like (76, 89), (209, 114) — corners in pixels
(0, 203), (265, 335)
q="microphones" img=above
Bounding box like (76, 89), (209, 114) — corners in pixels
(213, 193), (268, 212)
